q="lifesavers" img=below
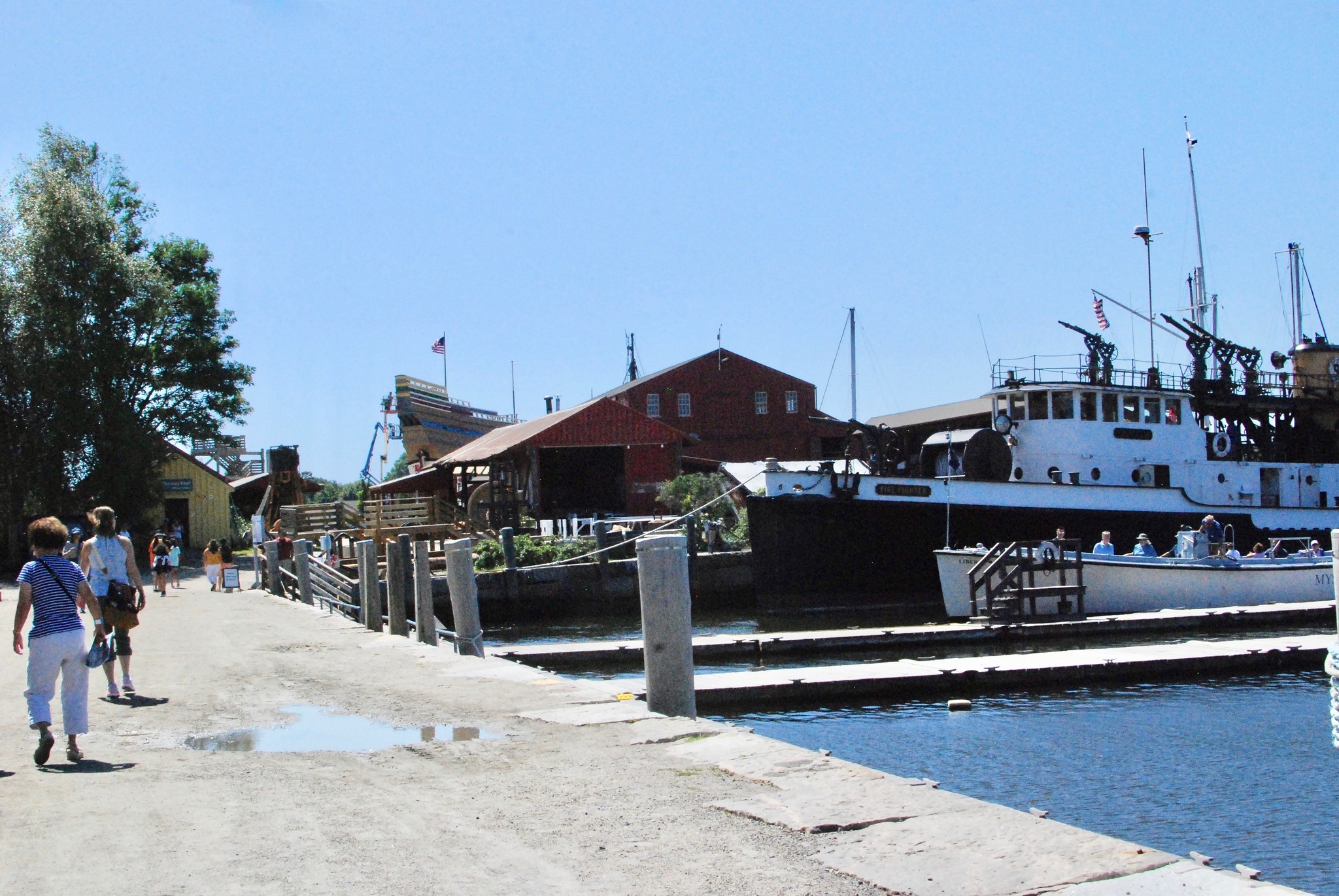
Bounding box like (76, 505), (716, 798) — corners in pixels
(1034, 541), (1060, 564)
(1212, 431), (1232, 458)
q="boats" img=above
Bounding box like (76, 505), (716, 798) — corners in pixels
(932, 426), (1333, 618)
(717, 114), (1334, 618)
(381, 330), (646, 507)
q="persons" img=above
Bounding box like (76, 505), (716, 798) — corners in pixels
(1052, 526), (1066, 540)
(258, 545), (269, 590)
(1123, 533), (1160, 558)
(324, 532), (338, 568)
(1264, 538), (1292, 558)
(273, 528), (294, 591)
(62, 506), (146, 697)
(1247, 543), (1265, 558)
(1159, 531), (1183, 557)
(1225, 542), (1240, 559)
(218, 538), (235, 592)
(1298, 540), (1326, 557)
(388, 391), (394, 410)
(13, 516), (107, 765)
(1191, 515), (1224, 556)
(204, 540), (224, 592)
(1093, 531), (1114, 555)
(149, 518), (183, 598)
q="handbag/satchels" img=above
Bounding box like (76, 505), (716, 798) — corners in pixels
(102, 580), (140, 630)
(86, 635), (111, 669)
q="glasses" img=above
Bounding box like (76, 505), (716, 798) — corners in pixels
(1059, 532), (1064, 534)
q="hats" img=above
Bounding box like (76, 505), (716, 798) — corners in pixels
(70, 528), (81, 534)
(1136, 533), (1149, 540)
(154, 530), (166, 537)
(1311, 540), (1319, 546)
(278, 528), (288, 534)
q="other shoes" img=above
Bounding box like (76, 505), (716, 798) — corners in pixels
(106, 684), (120, 698)
(210, 583), (216, 591)
(65, 745), (86, 761)
(34, 731), (55, 766)
(153, 589), (162, 592)
(176, 582), (180, 587)
(159, 590), (166, 597)
(122, 679), (135, 693)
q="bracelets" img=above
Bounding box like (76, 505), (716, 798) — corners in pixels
(94, 619), (104, 625)
(13, 631), (21, 634)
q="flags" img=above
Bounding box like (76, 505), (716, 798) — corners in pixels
(1093, 297), (1110, 331)
(431, 336), (444, 354)
(1185, 131), (1197, 158)
